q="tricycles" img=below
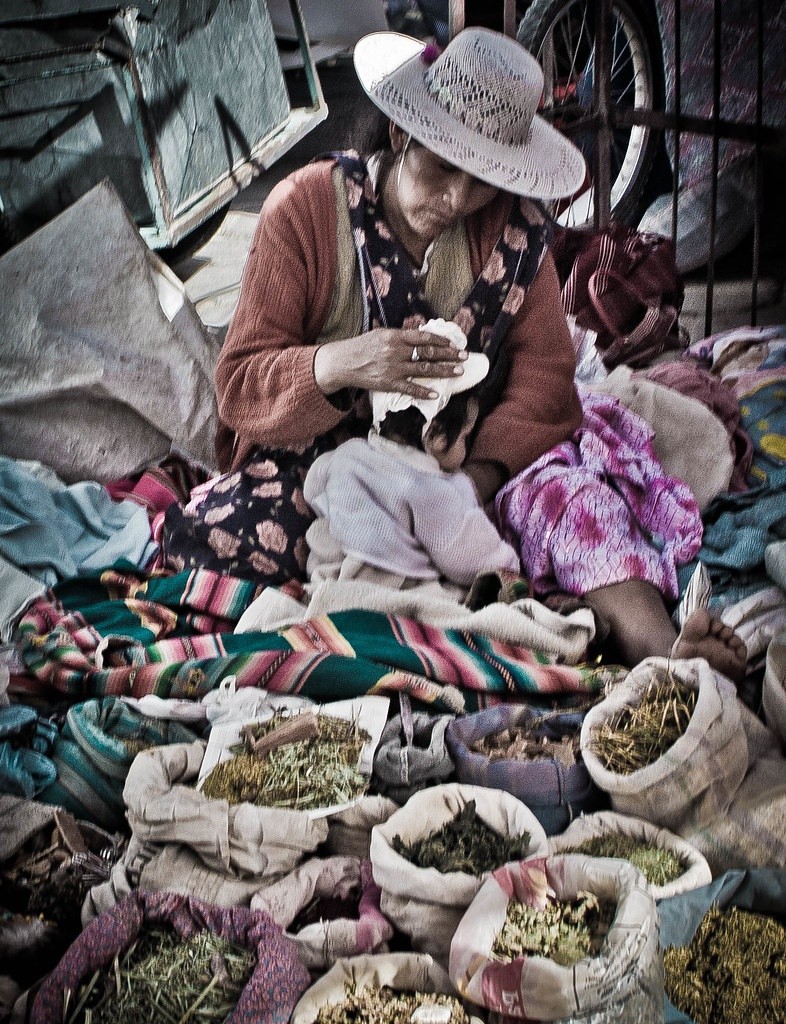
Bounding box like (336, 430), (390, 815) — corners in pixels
(0, 1), (333, 276)
(381, 0), (666, 248)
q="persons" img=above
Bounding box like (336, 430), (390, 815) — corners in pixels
(156, 26), (748, 682)
(303, 350), (519, 604)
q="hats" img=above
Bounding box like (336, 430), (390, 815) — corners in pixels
(351, 27), (587, 200)
(367, 349), (489, 408)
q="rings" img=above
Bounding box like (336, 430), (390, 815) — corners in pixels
(411, 347), (418, 361)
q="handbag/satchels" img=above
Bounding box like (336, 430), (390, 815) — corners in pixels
(553, 225), (681, 367)
